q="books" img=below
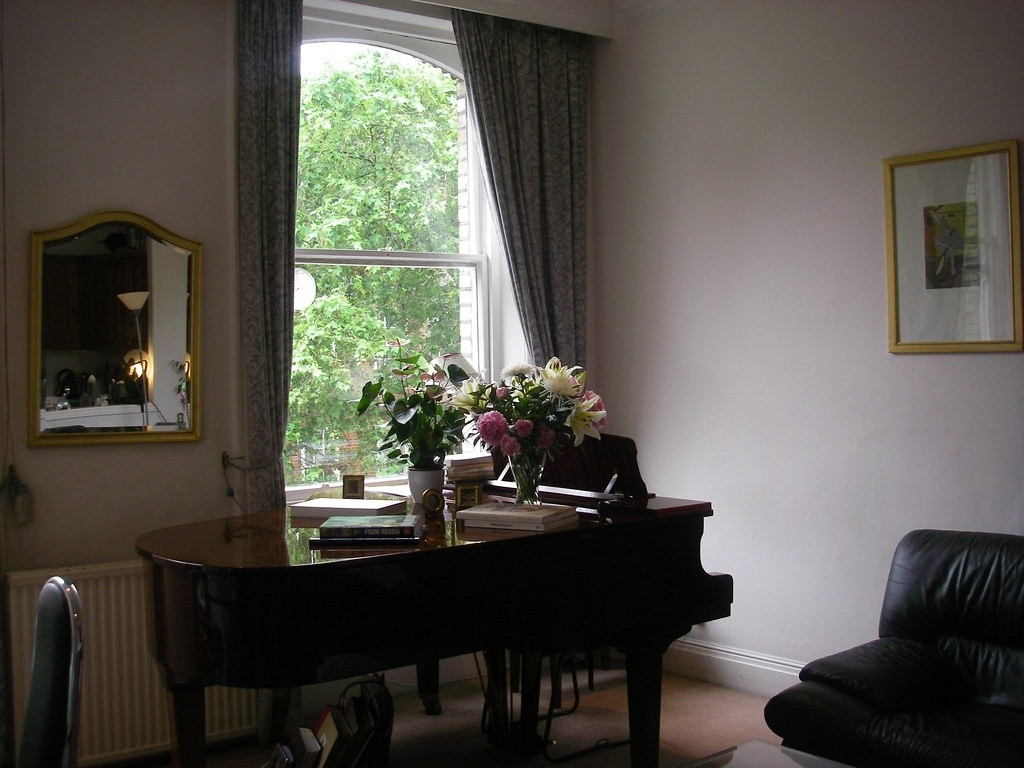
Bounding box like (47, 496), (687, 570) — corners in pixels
(320, 549), (417, 559)
(289, 498), (407, 517)
(309, 515), (427, 548)
(445, 453), (496, 481)
(289, 516), (328, 528)
(456, 503), (579, 531)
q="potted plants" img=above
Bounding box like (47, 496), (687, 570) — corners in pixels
(354, 342), (468, 505)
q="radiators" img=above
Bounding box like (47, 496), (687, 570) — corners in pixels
(5, 555), (261, 768)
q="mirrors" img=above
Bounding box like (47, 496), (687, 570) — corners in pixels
(27, 207), (209, 447)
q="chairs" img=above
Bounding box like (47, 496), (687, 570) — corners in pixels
(489, 430), (657, 695)
(19, 574), (86, 768)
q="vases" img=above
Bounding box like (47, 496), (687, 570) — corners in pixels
(506, 450), (547, 505)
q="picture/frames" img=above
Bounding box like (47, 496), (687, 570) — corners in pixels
(456, 482), (483, 511)
(882, 139), (1024, 354)
(342, 474), (364, 500)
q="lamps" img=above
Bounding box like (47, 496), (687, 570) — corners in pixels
(114, 291), (151, 430)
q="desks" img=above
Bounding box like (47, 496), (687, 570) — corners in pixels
(130, 495), (735, 768)
(39, 404), (161, 433)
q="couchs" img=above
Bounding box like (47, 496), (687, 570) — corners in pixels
(762, 528), (1024, 768)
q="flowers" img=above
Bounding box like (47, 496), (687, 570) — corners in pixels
(472, 355), (607, 458)
